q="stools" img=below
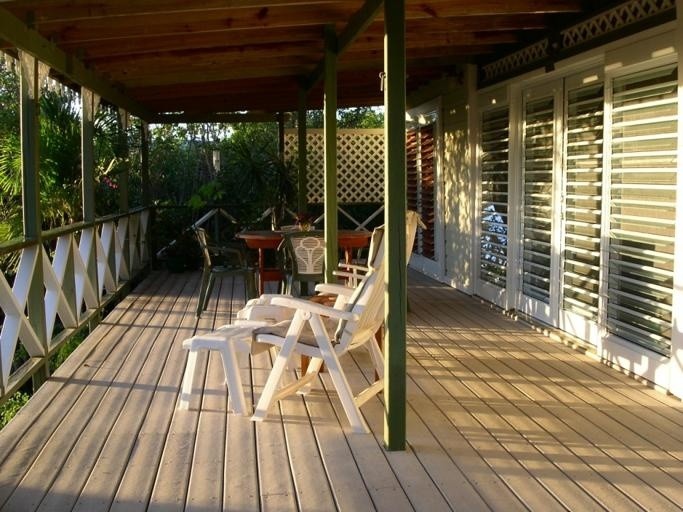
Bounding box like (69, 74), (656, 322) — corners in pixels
(176, 319), (274, 417)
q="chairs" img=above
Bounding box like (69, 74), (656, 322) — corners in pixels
(195, 210), (377, 310)
(233, 212), (419, 431)
